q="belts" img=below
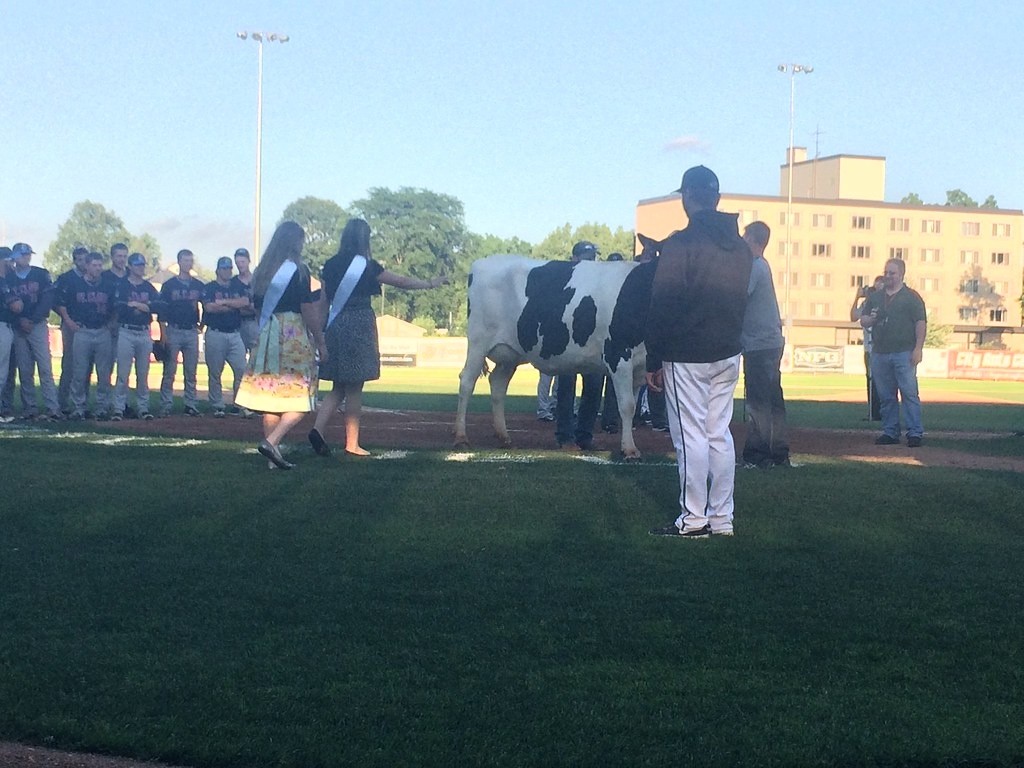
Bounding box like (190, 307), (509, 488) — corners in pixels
(76, 322), (106, 329)
(240, 316), (255, 321)
(122, 324), (149, 331)
(168, 324), (193, 330)
(211, 327), (238, 333)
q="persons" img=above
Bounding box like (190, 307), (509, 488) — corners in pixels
(645, 165), (754, 540)
(234, 221), (328, 470)
(308, 219), (449, 455)
(736, 220), (790, 467)
(850, 258), (927, 446)
(538, 241), (671, 449)
(0, 242), (255, 424)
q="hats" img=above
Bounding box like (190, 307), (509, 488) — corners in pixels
(0, 247), (14, 260)
(13, 243), (33, 255)
(572, 241), (597, 257)
(234, 248), (249, 257)
(672, 165), (719, 193)
(128, 253), (146, 265)
(217, 256), (232, 269)
(607, 253), (623, 261)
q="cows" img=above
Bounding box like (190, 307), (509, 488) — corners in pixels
(451, 255), (660, 460)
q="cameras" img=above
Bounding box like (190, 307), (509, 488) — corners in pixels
(862, 286), (874, 297)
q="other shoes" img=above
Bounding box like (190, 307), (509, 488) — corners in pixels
(742, 462), (761, 469)
(771, 458), (790, 469)
(863, 416), (878, 421)
(540, 408), (670, 451)
(308, 429), (331, 457)
(258, 439), (293, 469)
(268, 460), (296, 469)
(649, 524), (710, 539)
(706, 522), (734, 536)
(344, 449), (376, 456)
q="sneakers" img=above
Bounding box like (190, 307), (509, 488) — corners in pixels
(875, 435), (899, 444)
(0, 404), (264, 423)
(906, 436), (921, 447)
